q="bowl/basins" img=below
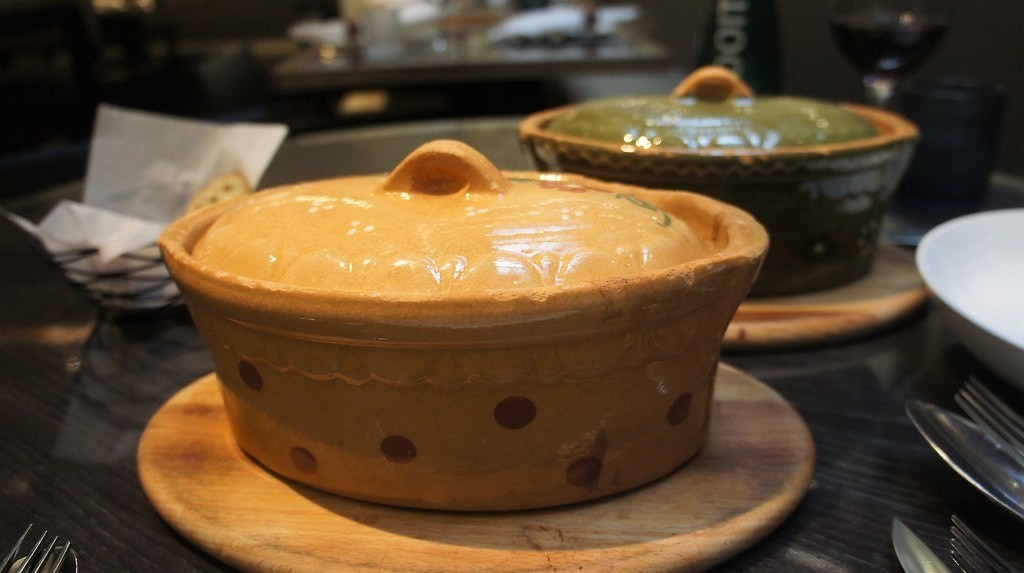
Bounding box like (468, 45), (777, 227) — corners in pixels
(914, 207), (1024, 392)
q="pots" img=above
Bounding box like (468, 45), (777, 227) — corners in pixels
(156, 138), (770, 513)
(517, 65), (920, 300)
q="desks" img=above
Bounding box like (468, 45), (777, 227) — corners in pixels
(0, 120), (1024, 573)
(270, 24), (671, 117)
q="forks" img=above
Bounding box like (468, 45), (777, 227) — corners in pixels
(0, 522), (71, 573)
(949, 514), (1018, 573)
(954, 376), (1024, 451)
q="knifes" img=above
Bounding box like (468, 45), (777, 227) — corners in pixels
(891, 517), (952, 573)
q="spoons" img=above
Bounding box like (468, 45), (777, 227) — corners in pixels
(8, 546), (78, 573)
(903, 399), (1024, 519)
